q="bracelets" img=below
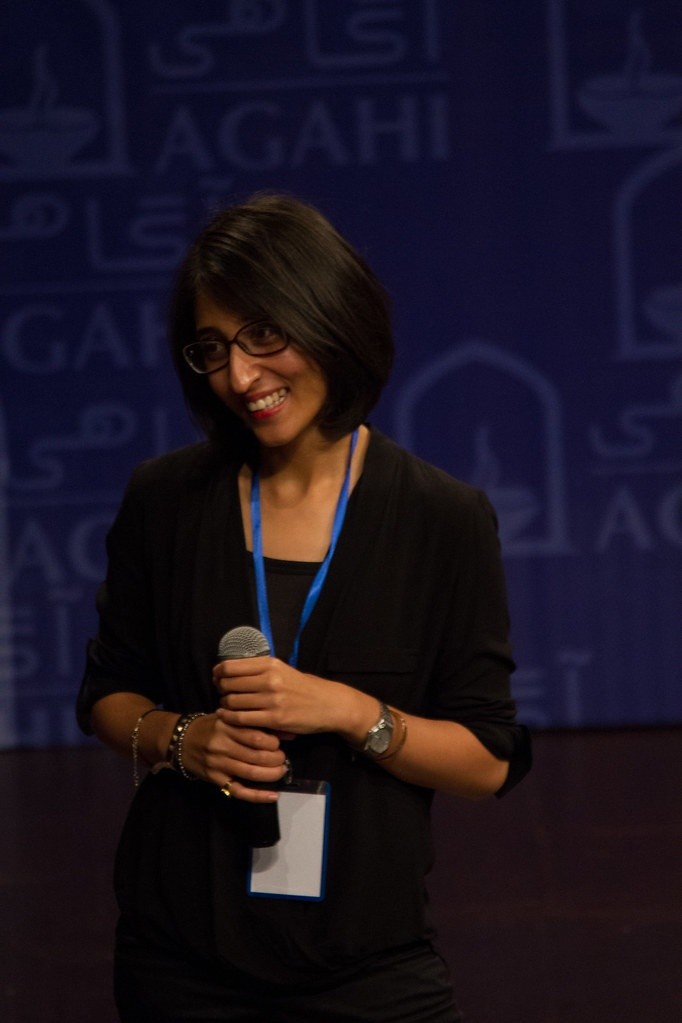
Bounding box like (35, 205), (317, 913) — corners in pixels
(372, 712), (407, 762)
(131, 708), (160, 789)
(168, 711), (207, 784)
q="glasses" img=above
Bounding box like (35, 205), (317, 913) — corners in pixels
(182, 318), (291, 375)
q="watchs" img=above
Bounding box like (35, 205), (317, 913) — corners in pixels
(361, 703), (393, 757)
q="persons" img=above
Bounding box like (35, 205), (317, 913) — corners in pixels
(72, 196), (533, 1023)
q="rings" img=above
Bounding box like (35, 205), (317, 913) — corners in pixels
(220, 778), (235, 797)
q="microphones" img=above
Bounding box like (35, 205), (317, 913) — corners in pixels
(216, 626), (281, 848)
